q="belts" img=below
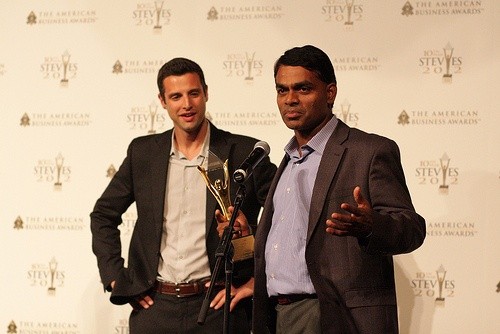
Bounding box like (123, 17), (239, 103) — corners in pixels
(271, 293), (308, 306)
(153, 276), (227, 299)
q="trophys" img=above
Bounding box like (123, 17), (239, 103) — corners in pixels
(196, 150), (257, 263)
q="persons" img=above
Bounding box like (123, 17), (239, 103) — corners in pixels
(88, 57), (278, 333)
(214, 46), (425, 334)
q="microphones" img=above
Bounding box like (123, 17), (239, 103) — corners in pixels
(233, 141), (270, 184)
